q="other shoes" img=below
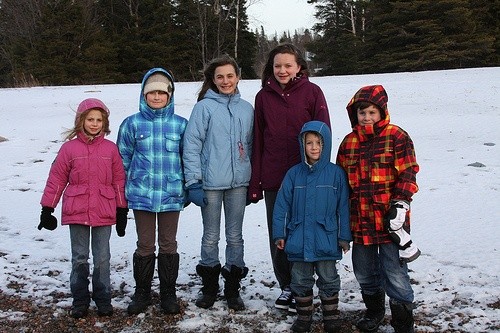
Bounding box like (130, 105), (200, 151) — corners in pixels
(98, 302), (114, 317)
(72, 305), (88, 318)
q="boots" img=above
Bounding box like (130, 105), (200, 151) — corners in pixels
(127, 252), (156, 314)
(291, 295), (314, 333)
(321, 296), (341, 333)
(157, 253), (180, 313)
(195, 263), (221, 309)
(389, 300), (414, 333)
(359, 291), (385, 331)
(221, 265), (249, 311)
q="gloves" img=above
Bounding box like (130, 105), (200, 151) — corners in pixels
(116, 207), (128, 237)
(38, 211), (57, 231)
(387, 226), (421, 263)
(385, 201), (409, 231)
(184, 180), (208, 207)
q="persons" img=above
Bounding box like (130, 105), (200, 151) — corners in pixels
(117, 68), (188, 315)
(181, 54), (255, 311)
(38, 99), (130, 317)
(336, 84), (419, 333)
(246, 43), (331, 312)
(272, 121), (350, 333)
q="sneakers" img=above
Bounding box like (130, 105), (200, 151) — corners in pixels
(275, 289), (292, 309)
(289, 297), (297, 312)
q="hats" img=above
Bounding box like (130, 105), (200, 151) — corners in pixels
(144, 72), (172, 105)
